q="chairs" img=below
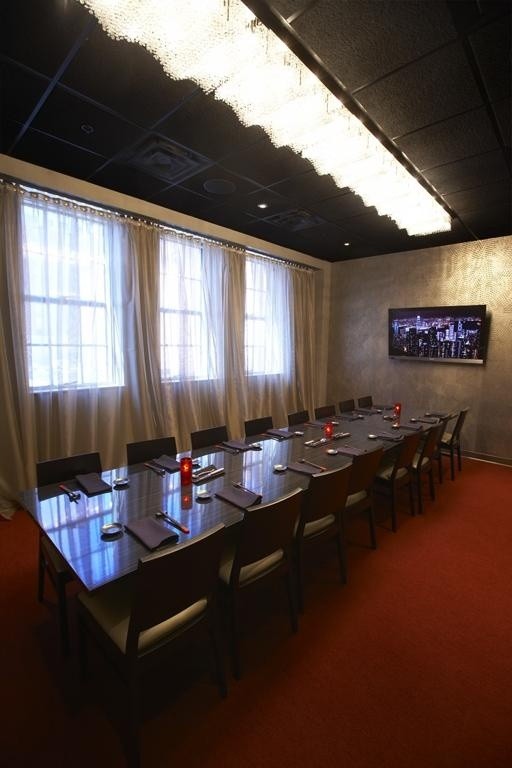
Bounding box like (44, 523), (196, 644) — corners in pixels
(315, 404), (337, 419)
(214, 487), (304, 631)
(338, 399), (355, 412)
(243, 415), (274, 437)
(287, 410), (310, 426)
(340, 443), (386, 585)
(190, 424), (228, 450)
(36, 450), (101, 487)
(358, 396), (372, 409)
(77, 524), (225, 675)
(125, 437), (178, 465)
(289, 462), (354, 614)
(365, 406), (471, 550)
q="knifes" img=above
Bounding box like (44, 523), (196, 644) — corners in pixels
(197, 467), (225, 483)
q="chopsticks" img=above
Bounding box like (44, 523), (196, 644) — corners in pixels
(156, 508), (189, 533)
(58, 484), (74, 495)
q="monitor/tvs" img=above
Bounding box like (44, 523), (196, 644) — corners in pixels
(389, 305), (486, 364)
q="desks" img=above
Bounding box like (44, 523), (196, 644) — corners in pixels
(15, 404), (451, 666)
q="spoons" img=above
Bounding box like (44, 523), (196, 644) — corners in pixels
(191, 469), (215, 479)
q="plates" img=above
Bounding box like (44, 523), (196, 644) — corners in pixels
(331, 421), (338, 424)
(295, 431), (304, 435)
(274, 464), (287, 472)
(327, 449), (338, 454)
(101, 523), (123, 535)
(369, 433), (378, 440)
(197, 490), (211, 500)
(251, 443), (260, 448)
(192, 460), (203, 465)
(113, 477), (128, 487)
